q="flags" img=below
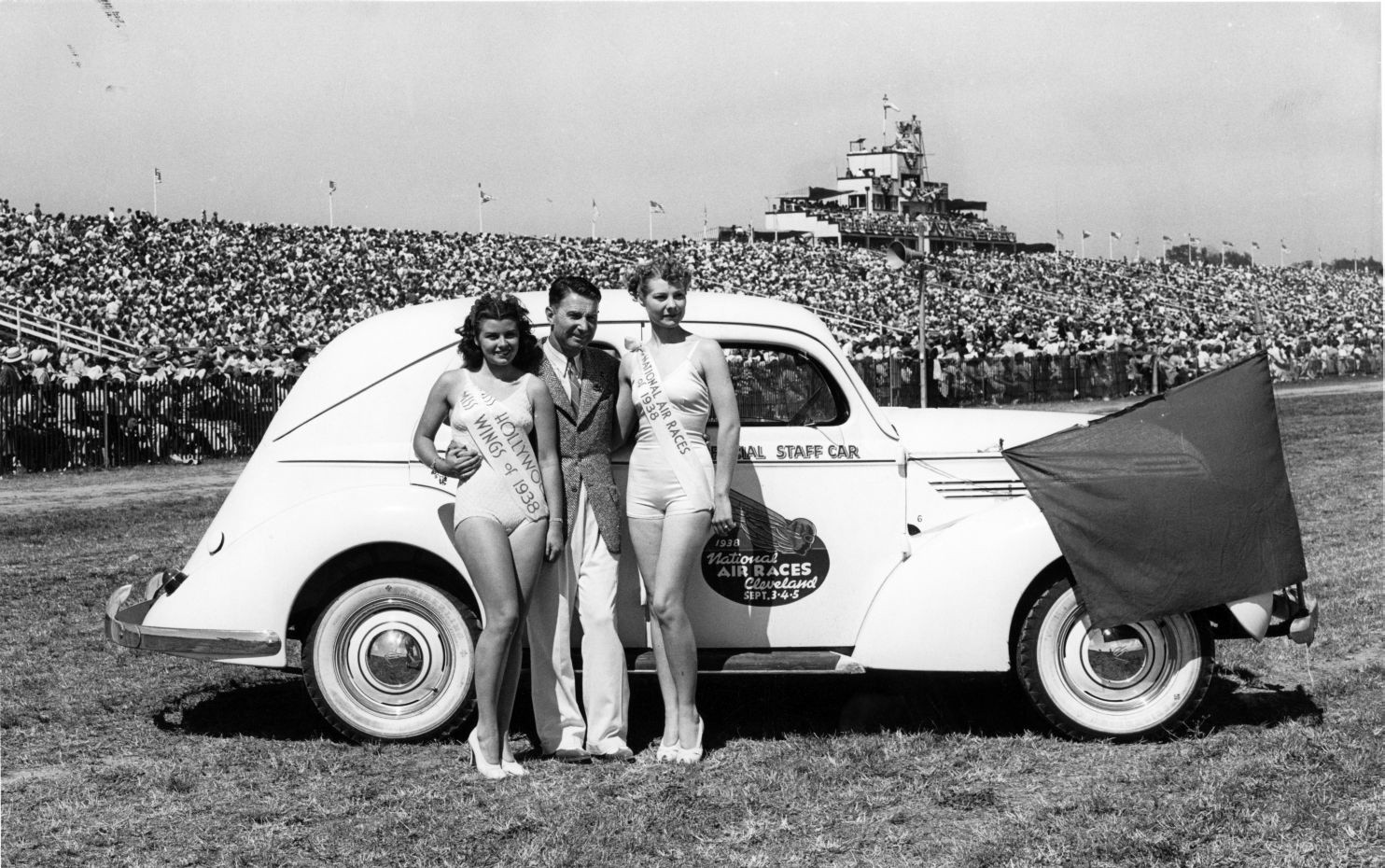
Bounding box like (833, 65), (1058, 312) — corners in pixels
(1003, 344), (1310, 632)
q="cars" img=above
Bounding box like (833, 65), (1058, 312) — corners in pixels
(102, 288), (1326, 741)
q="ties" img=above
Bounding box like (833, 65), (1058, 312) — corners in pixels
(566, 360), (583, 420)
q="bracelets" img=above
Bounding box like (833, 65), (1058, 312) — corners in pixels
(431, 458), (448, 485)
(549, 517), (564, 523)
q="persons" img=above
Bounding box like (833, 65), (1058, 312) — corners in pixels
(447, 274), (714, 761)
(605, 253), (741, 765)
(413, 293), (565, 782)
(1, 112), (1384, 463)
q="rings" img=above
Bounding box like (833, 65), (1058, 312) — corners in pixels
(724, 524), (729, 530)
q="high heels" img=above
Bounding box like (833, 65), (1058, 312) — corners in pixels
(467, 727), (507, 779)
(500, 760), (530, 777)
(676, 715), (704, 765)
(657, 736), (679, 763)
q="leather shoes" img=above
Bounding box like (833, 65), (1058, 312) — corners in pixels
(543, 749), (591, 762)
(601, 753), (636, 763)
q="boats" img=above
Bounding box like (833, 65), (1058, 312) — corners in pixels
(693, 94), (1017, 257)
